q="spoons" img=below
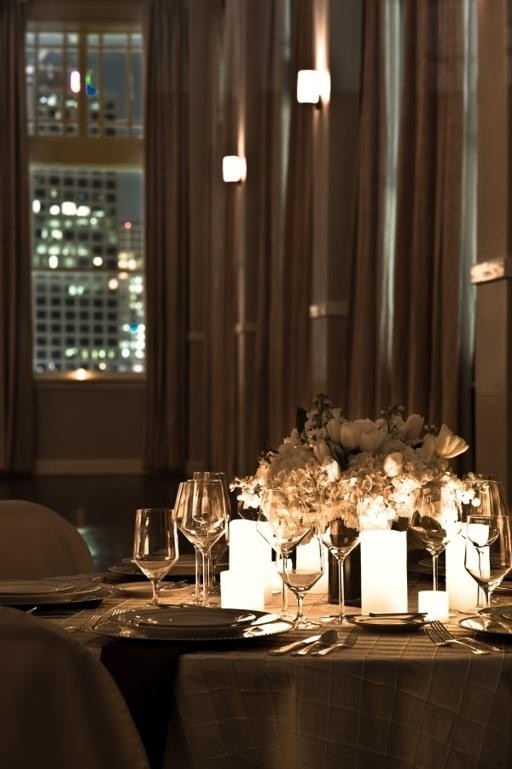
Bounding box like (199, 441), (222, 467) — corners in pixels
(298, 627), (338, 656)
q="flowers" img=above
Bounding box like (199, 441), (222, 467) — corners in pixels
(229, 396), (470, 532)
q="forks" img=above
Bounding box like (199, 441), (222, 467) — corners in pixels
(423, 621), (507, 654)
(317, 635), (358, 657)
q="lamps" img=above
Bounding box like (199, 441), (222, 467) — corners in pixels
(296, 70), (322, 109)
(222, 155), (242, 184)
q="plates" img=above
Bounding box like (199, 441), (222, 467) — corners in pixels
(108, 579), (282, 638)
(1, 579), (102, 602)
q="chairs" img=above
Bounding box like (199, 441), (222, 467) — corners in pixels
(0, 499), (151, 769)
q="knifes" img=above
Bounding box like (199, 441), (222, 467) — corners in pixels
(267, 634), (321, 655)
